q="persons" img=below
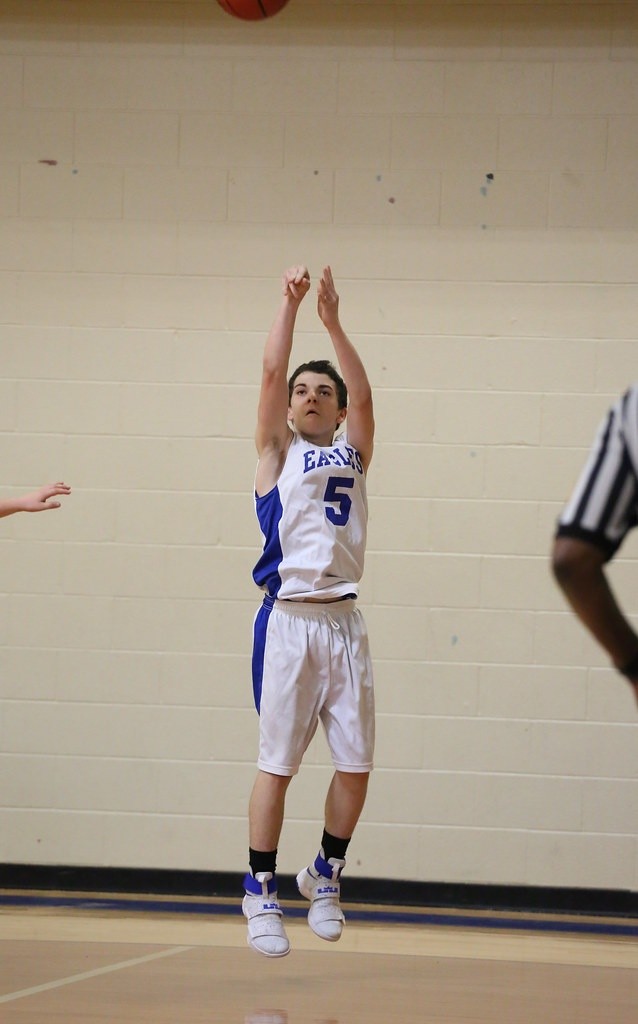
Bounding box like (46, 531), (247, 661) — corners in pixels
(240, 264), (375, 958)
(552, 383), (638, 704)
(0, 481), (72, 520)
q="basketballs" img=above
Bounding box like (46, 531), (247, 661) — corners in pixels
(216, 0), (290, 20)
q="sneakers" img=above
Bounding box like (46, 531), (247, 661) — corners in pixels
(241, 872), (290, 959)
(298, 850), (346, 939)
